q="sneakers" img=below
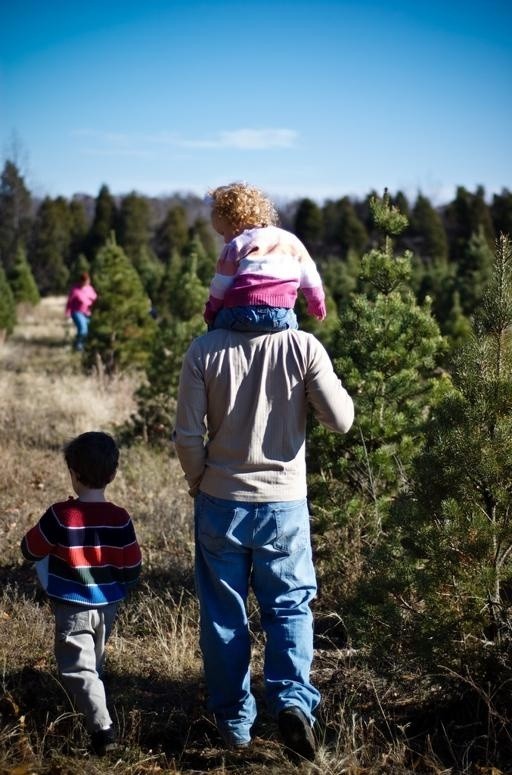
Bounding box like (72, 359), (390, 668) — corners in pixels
(93, 727), (117, 752)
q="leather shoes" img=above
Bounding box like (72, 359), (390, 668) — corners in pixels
(278, 706), (316, 766)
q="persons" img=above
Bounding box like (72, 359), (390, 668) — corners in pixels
(173, 327), (357, 761)
(147, 299), (162, 324)
(19, 432), (143, 754)
(65, 271), (98, 350)
(201, 180), (328, 335)
